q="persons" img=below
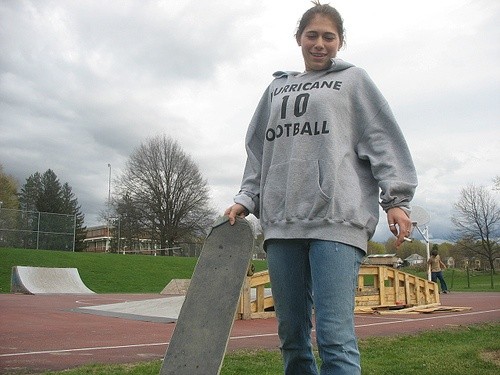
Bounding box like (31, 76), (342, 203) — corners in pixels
(428, 249), (450, 294)
(223, 3), (418, 375)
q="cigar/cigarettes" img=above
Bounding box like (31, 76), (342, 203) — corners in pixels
(403, 237), (412, 243)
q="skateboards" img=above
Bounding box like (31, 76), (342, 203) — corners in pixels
(158, 215), (255, 375)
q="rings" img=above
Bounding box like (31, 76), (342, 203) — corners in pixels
(406, 229), (410, 233)
(389, 224), (394, 227)
